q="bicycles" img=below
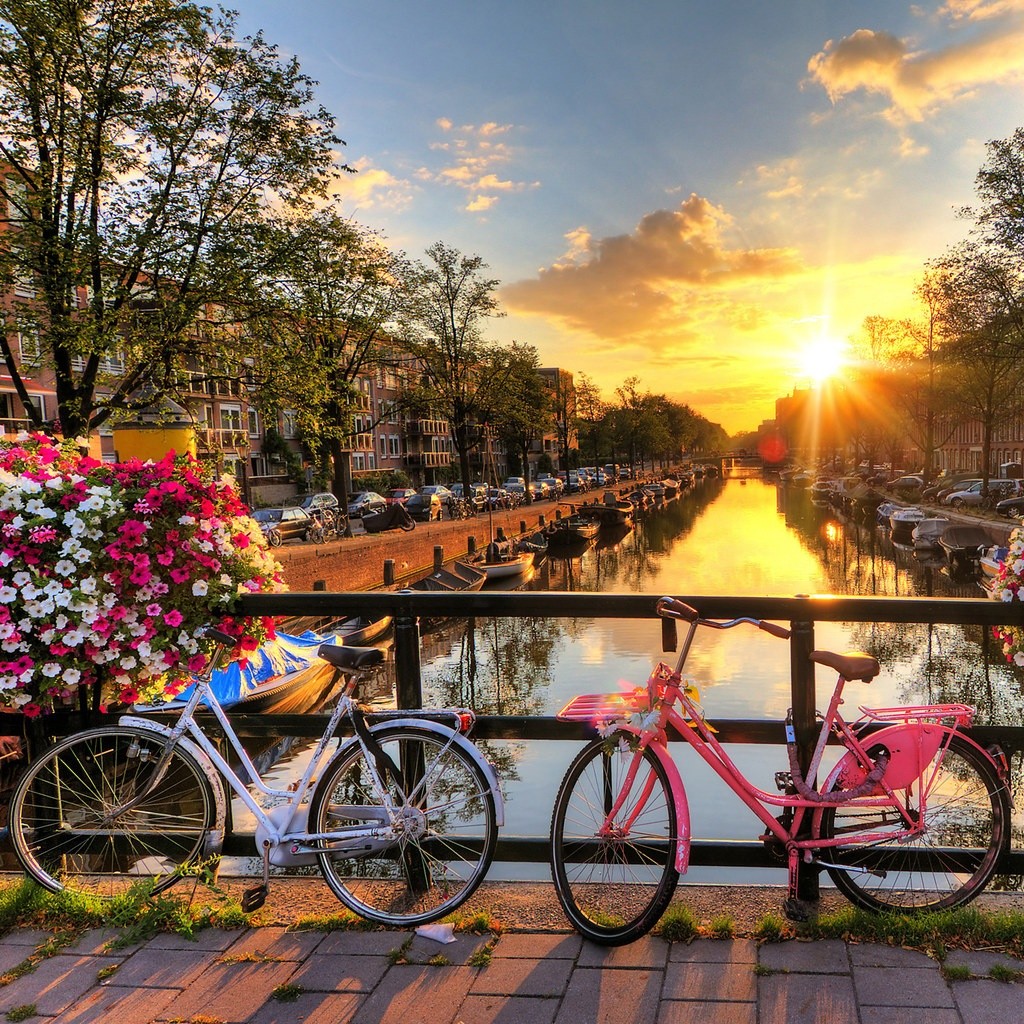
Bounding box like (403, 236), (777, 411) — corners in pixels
(549, 594), (1018, 949)
(306, 505), (350, 545)
(6, 629), (506, 928)
(253, 515), (285, 547)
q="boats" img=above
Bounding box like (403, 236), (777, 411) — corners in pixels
(407, 561), (485, 593)
(301, 615), (392, 649)
(934, 523), (997, 567)
(909, 515), (949, 549)
(780, 455), (900, 513)
(554, 513), (603, 540)
(568, 496), (634, 527)
(876, 501), (901, 524)
(516, 531), (549, 558)
(104, 627), (350, 749)
(887, 506), (927, 532)
(977, 545), (1014, 579)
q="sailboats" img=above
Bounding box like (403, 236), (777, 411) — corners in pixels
(464, 420), (535, 579)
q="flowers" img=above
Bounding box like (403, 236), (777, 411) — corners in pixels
(988, 515), (1024, 667)
(0, 430), (288, 718)
(598, 687), (660, 758)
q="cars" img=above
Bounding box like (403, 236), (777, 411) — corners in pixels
(341, 491), (388, 520)
(885, 475), (922, 492)
(936, 479), (983, 506)
(905, 472), (924, 480)
(247, 505), (313, 543)
(922, 470), (997, 503)
(944, 479), (1023, 508)
(419, 463), (718, 520)
(384, 488), (418, 509)
(402, 492), (442, 525)
(283, 491), (339, 517)
(995, 495), (1024, 519)
